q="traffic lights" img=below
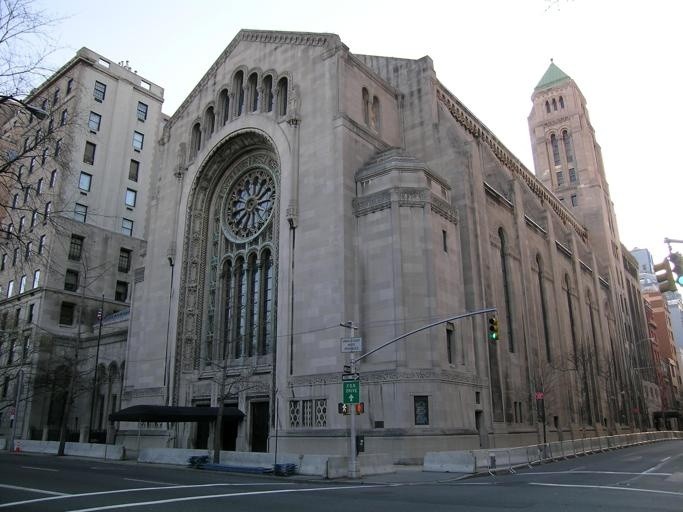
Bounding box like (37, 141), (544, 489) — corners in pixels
(670, 252), (683, 287)
(488, 314), (499, 340)
(653, 257), (677, 293)
(338, 403), (364, 413)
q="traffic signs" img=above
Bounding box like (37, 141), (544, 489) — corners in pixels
(341, 373), (359, 381)
(342, 381), (359, 404)
(341, 337), (362, 352)
(342, 366), (356, 373)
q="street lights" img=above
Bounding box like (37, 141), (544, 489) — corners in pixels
(0, 329), (24, 452)
(87, 293), (106, 443)
(628, 336), (657, 434)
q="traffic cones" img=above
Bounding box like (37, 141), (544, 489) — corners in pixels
(15, 440), (21, 452)
